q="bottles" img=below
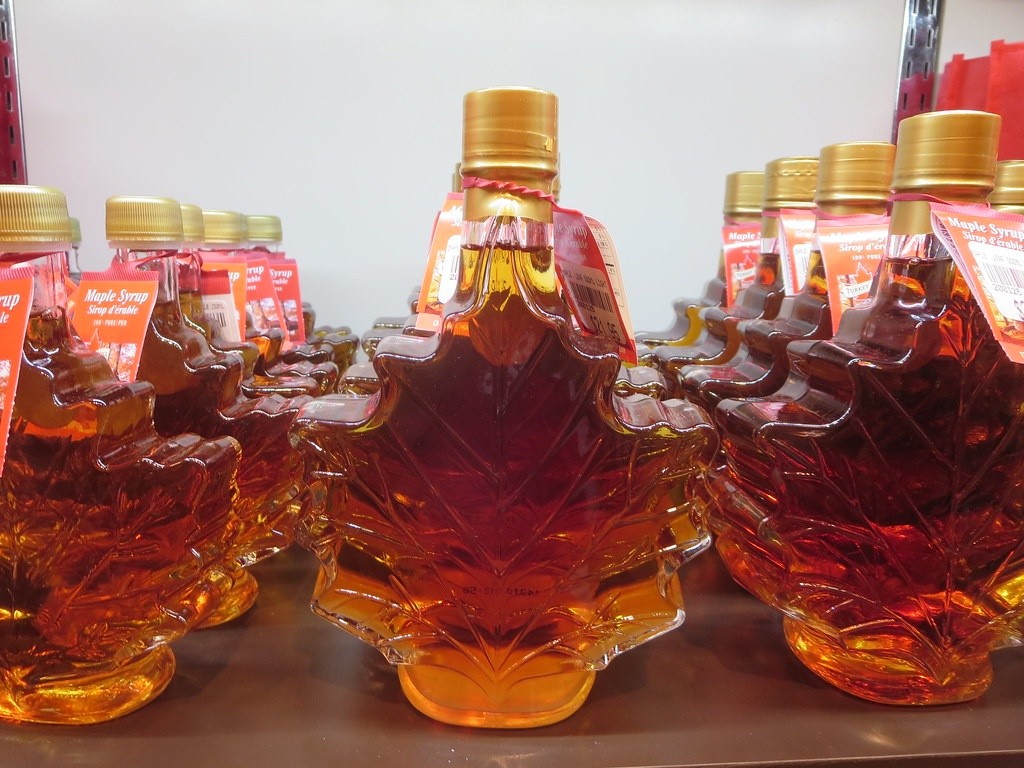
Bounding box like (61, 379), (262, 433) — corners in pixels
(0, 85), (1024, 729)
